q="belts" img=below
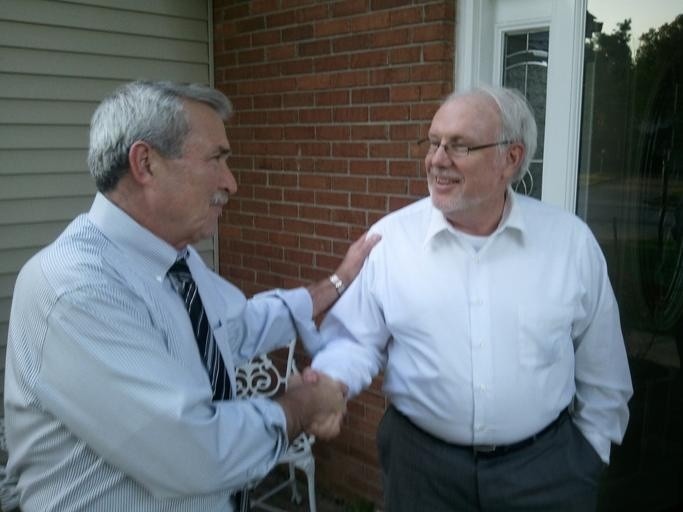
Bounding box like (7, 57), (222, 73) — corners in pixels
(392, 405), (577, 459)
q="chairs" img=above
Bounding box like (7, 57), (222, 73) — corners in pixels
(235, 335), (318, 509)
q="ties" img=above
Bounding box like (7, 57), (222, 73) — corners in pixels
(166, 258), (253, 511)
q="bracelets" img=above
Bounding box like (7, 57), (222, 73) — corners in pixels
(328, 272), (345, 295)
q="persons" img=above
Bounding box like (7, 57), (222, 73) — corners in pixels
(301, 89), (634, 512)
(3, 78), (382, 512)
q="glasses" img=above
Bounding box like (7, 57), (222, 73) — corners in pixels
(417, 138), (516, 158)
